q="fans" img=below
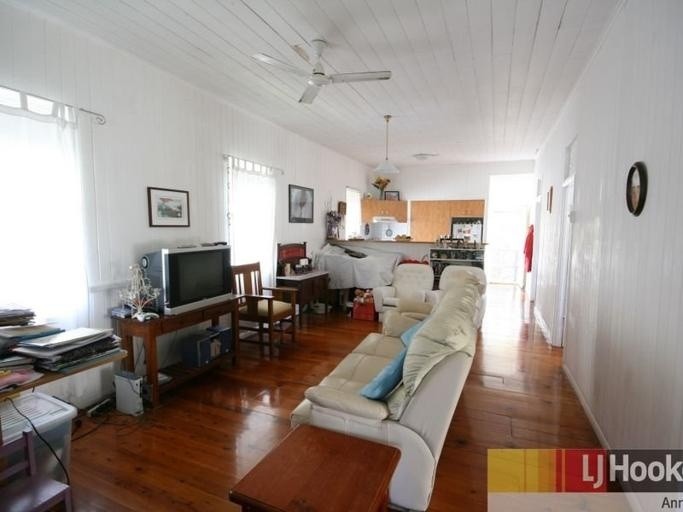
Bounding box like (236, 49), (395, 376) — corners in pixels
(250, 39), (393, 106)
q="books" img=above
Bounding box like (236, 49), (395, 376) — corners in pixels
(0, 321), (123, 392)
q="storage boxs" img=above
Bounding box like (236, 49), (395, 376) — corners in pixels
(1, 391), (79, 484)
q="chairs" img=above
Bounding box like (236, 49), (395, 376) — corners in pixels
(277, 239), (307, 260)
(0, 429), (74, 512)
(230, 261), (298, 360)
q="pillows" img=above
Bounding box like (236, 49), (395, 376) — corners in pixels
(445, 269), (480, 290)
(359, 350), (406, 400)
(382, 309), (418, 338)
(402, 285), (482, 396)
(400, 320), (424, 348)
(398, 288), (425, 314)
(304, 386), (389, 421)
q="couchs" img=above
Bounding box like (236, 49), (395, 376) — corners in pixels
(288, 279), (486, 509)
(372, 262), (434, 323)
(425, 264), (487, 331)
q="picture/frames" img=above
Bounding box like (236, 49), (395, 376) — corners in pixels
(287, 183), (315, 224)
(384, 191), (399, 201)
(625, 159), (649, 216)
(146, 185), (192, 231)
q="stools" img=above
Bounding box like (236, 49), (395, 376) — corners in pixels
(228, 421), (402, 512)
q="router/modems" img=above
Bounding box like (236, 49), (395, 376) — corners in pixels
(114, 372), (145, 417)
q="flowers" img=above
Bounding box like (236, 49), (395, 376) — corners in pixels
(369, 174), (393, 200)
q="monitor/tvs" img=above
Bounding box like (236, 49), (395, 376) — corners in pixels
(142, 244), (233, 316)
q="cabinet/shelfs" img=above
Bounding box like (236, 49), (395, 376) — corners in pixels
(361, 198), (408, 224)
(0, 347), (128, 412)
(276, 271), (330, 329)
(429, 248), (485, 291)
(411, 199), (484, 243)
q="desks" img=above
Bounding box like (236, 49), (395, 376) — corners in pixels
(111, 294), (246, 407)
